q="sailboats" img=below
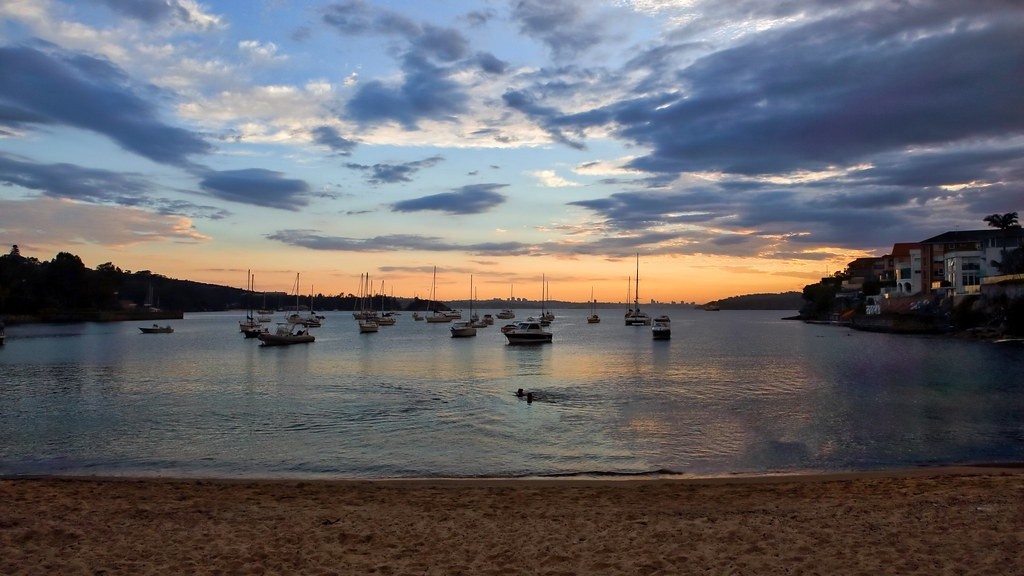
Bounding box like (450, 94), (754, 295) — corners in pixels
(623, 252), (652, 327)
(585, 288), (601, 324)
(236, 264), (557, 338)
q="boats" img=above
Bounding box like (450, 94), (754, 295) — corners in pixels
(449, 319), (477, 337)
(503, 320), (553, 345)
(651, 314), (672, 340)
(704, 306), (720, 311)
(137, 324), (175, 333)
(257, 321), (316, 345)
